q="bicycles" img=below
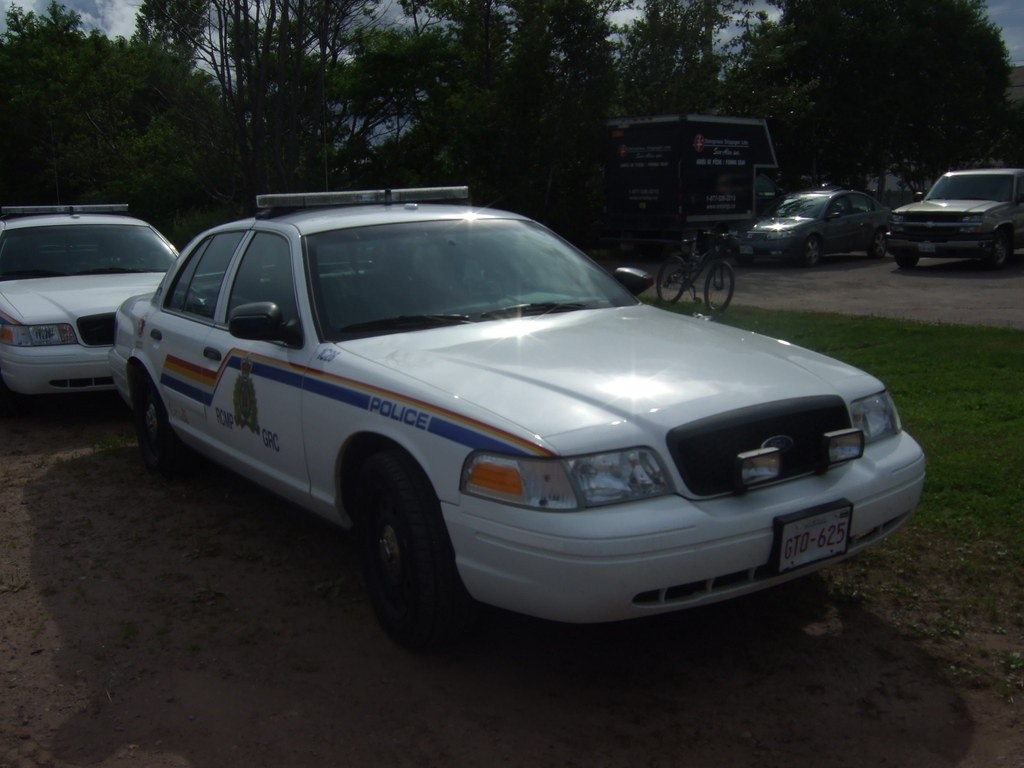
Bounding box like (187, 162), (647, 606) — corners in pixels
(656, 231), (744, 313)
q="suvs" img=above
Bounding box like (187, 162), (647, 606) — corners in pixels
(884, 168), (1024, 273)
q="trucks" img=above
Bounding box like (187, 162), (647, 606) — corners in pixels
(599, 113), (785, 257)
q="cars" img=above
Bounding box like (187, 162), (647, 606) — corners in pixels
(732, 187), (893, 269)
(108, 184), (928, 656)
(0, 203), (187, 414)
